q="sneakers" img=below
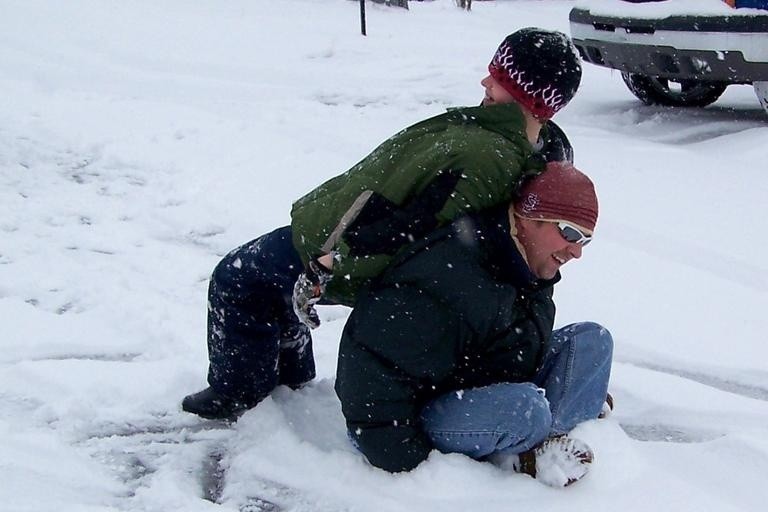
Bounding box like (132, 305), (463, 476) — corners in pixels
(513, 437), (594, 486)
(598, 393), (614, 419)
(182, 386), (245, 421)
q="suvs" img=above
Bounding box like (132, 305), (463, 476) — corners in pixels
(565, 0), (768, 119)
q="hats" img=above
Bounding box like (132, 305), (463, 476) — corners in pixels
(489, 27), (581, 117)
(513, 161), (598, 236)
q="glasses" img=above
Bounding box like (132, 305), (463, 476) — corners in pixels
(558, 223), (591, 246)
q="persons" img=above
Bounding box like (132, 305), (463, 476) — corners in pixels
(335, 161), (614, 487)
(181, 25), (582, 419)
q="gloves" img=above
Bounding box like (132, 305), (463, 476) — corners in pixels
(291, 257), (333, 330)
